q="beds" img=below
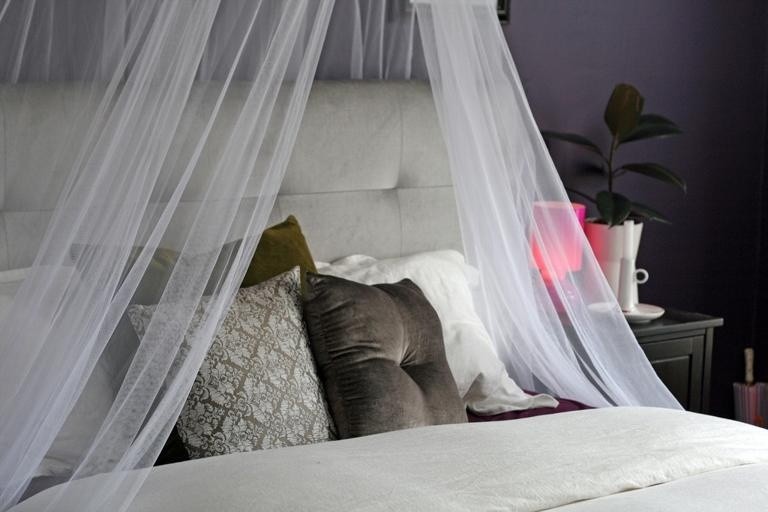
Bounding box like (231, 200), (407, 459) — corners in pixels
(1, 76), (768, 510)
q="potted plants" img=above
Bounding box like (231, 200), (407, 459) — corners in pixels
(541, 87), (685, 318)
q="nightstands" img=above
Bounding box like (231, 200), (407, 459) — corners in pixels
(624, 307), (722, 413)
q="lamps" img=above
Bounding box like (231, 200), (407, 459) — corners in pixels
(528, 197), (584, 315)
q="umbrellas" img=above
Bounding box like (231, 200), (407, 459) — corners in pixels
(731, 347), (766, 429)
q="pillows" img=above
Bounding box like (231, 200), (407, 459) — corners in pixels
(69, 215), (318, 466)
(330, 249), (560, 416)
(125, 264), (335, 460)
(1, 261), (119, 481)
(306, 270), (470, 440)
